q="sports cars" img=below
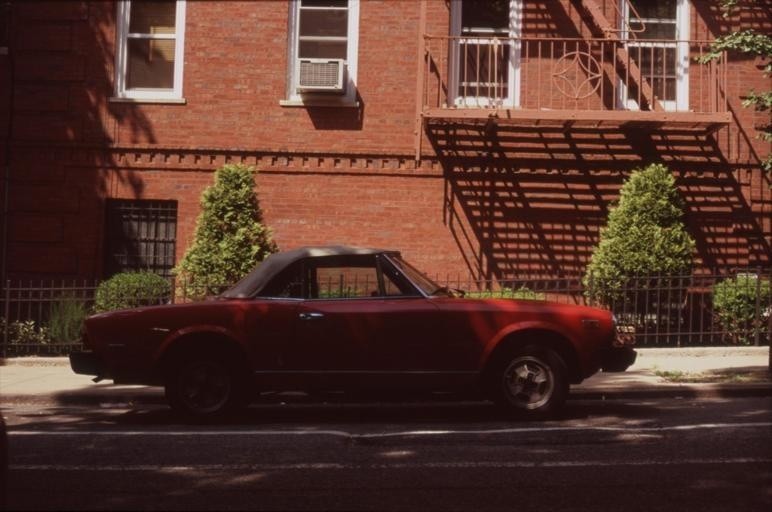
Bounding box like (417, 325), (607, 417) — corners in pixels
(70, 245), (636, 421)
